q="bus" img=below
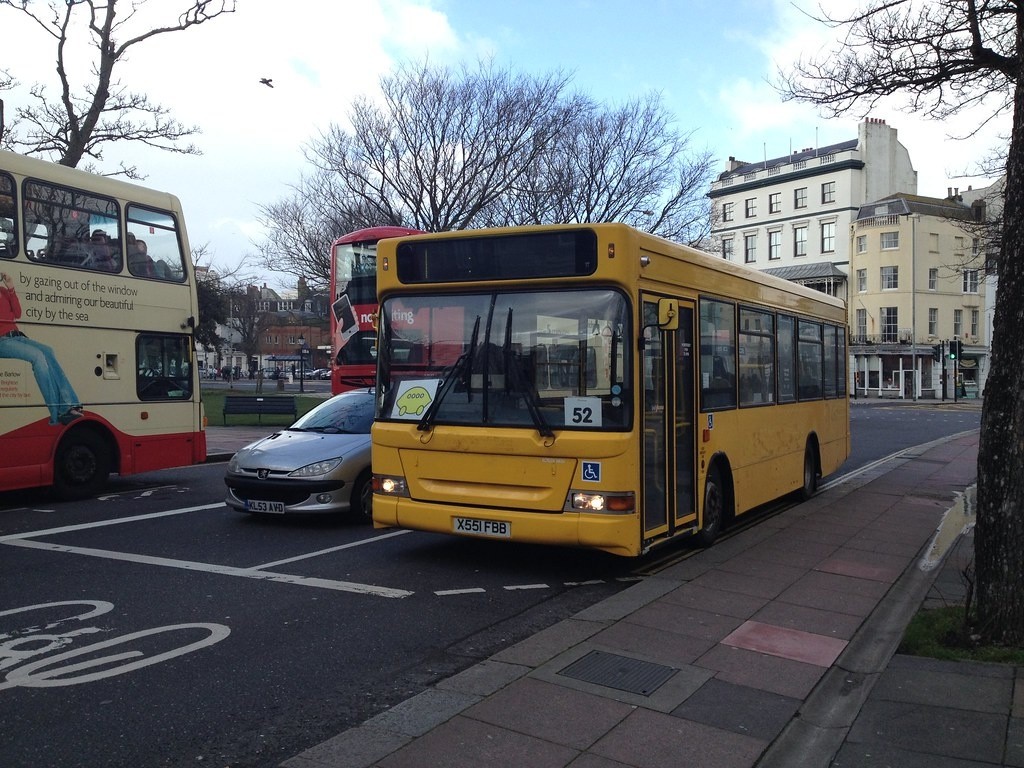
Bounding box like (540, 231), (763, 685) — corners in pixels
(371, 223), (852, 573)
(330, 227), (655, 405)
(0, 148), (209, 502)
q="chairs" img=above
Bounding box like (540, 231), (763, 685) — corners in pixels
(0, 244), (177, 280)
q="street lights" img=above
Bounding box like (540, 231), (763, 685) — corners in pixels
(297, 332), (306, 393)
(229, 275), (258, 390)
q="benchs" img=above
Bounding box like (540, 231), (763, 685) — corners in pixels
(223, 395), (298, 427)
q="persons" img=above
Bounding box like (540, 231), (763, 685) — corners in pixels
(0, 272), (85, 426)
(649, 346), (846, 401)
(207, 363), (295, 381)
(89, 229), (180, 281)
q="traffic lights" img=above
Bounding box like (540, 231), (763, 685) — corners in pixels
(931, 345), (940, 362)
(949, 340), (964, 360)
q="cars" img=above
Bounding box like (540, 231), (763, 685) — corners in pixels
(224, 386), (388, 523)
(199, 367), (332, 380)
